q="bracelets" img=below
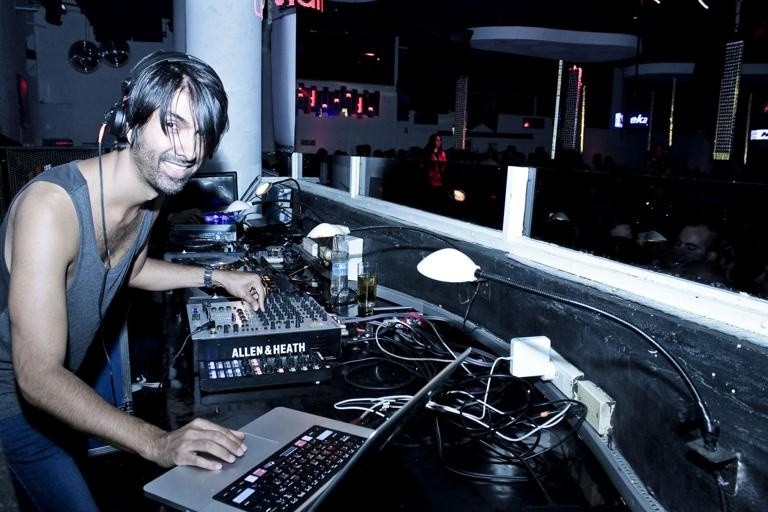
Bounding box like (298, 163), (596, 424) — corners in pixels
(203, 267), (213, 288)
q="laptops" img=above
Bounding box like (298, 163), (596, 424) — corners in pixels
(141, 344), (475, 512)
(159, 171), (240, 268)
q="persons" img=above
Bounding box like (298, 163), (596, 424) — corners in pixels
(0, 55), (269, 512)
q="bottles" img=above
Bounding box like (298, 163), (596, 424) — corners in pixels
(329, 234), (350, 297)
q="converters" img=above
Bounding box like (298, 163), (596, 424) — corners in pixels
(505, 334), (552, 381)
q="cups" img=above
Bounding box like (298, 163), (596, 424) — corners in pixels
(356, 261), (379, 308)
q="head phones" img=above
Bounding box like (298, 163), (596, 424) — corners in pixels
(106, 48), (219, 143)
